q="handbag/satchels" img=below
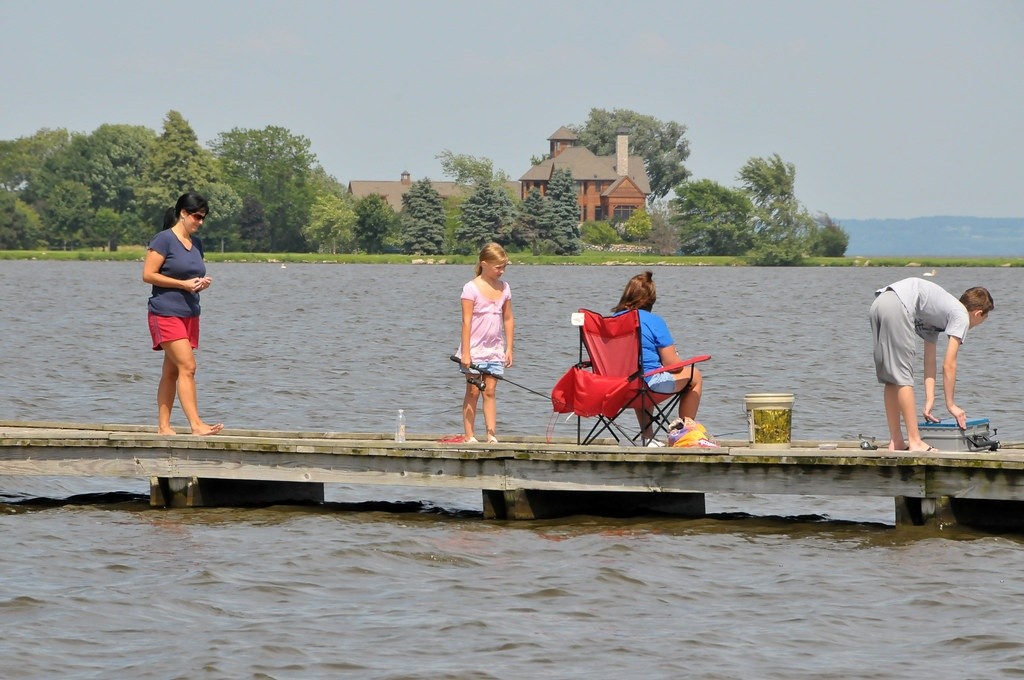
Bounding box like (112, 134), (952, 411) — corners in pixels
(667, 416), (721, 448)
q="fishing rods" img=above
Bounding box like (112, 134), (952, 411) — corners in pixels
(450, 355), (638, 432)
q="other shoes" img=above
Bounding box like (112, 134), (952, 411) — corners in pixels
(463, 437), (479, 443)
(487, 436), (498, 444)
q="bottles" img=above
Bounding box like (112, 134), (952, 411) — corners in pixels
(395, 409), (406, 444)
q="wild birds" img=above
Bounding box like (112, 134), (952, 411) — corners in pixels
(280, 263), (286, 269)
(922, 268), (936, 277)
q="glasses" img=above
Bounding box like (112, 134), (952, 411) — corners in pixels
(188, 214), (205, 220)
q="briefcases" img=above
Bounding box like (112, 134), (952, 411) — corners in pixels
(918, 417), (1001, 452)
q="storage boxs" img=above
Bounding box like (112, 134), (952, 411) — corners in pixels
(918, 417), (990, 452)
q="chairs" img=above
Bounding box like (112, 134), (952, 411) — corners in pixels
(573, 308), (711, 447)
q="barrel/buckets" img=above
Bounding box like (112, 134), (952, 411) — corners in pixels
(743, 392), (795, 451)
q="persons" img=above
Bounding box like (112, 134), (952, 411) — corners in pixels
(142, 191), (225, 436)
(454, 243), (515, 442)
(610, 271), (703, 448)
(868, 278), (996, 453)
